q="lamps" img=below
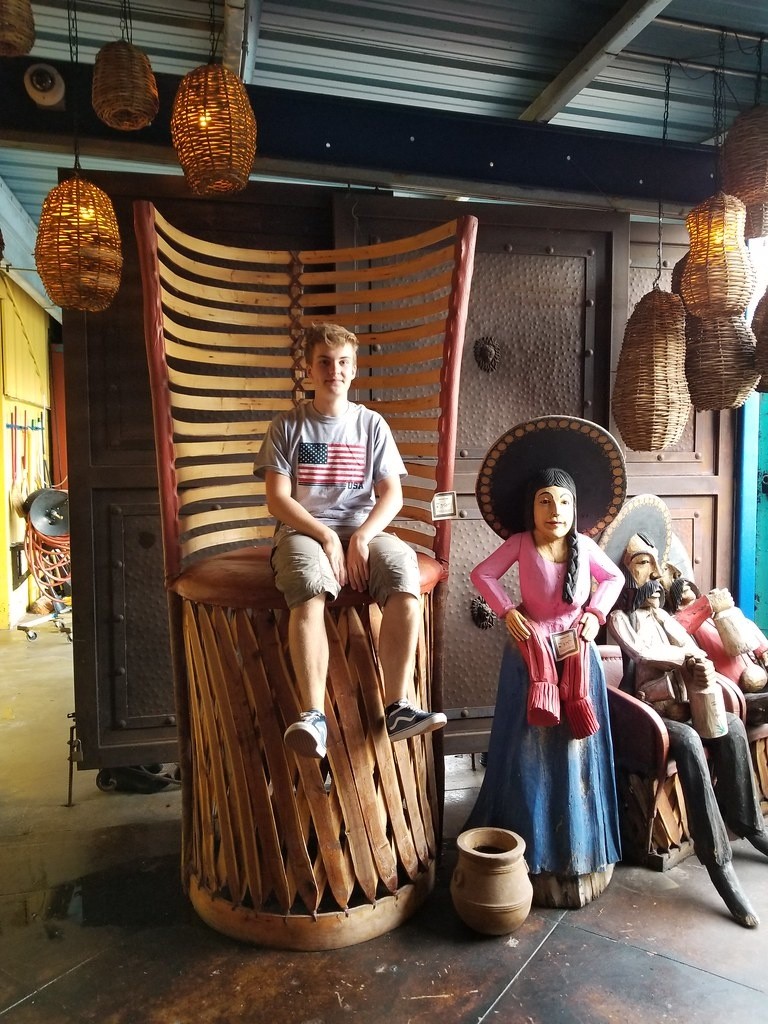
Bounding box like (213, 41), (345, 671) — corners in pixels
(34, 178), (124, 312)
(23, 62), (69, 113)
(169, 63), (258, 199)
(681, 190), (755, 317)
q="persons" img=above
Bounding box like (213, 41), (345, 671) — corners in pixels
(462, 468), (625, 910)
(602, 531), (768, 930)
(648, 559), (768, 732)
(253, 321), (449, 761)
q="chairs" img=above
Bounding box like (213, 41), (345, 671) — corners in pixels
(593, 643), (768, 872)
(129, 199), (480, 950)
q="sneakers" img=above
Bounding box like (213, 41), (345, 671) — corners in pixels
(282, 708), (329, 760)
(385, 704), (447, 743)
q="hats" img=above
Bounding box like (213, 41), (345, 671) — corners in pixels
(474, 414), (628, 547)
(601, 494), (672, 614)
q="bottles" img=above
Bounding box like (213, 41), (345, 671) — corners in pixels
(687, 654), (728, 739)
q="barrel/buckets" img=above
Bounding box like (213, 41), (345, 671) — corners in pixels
(450, 827), (533, 936)
